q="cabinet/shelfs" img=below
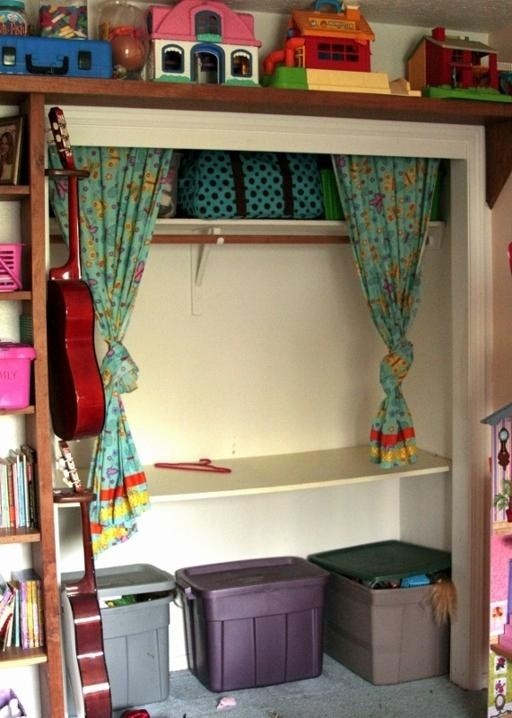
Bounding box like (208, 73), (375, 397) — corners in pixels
(0, 90), (64, 718)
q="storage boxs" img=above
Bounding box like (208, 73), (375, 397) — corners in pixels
(307, 540), (450, 686)
(61, 563), (176, 710)
(174, 557), (329, 693)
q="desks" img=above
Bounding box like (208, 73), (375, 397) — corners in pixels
(53, 444), (452, 592)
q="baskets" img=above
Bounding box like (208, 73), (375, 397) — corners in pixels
(0, 243), (23, 292)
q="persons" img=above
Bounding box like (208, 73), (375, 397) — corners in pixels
(1, 131), (15, 183)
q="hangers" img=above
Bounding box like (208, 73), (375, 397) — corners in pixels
(154, 458), (232, 474)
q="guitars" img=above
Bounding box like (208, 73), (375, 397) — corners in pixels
(45, 107), (105, 441)
(52, 441), (112, 718)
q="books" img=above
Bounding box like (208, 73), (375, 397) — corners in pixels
(0, 443), (47, 653)
